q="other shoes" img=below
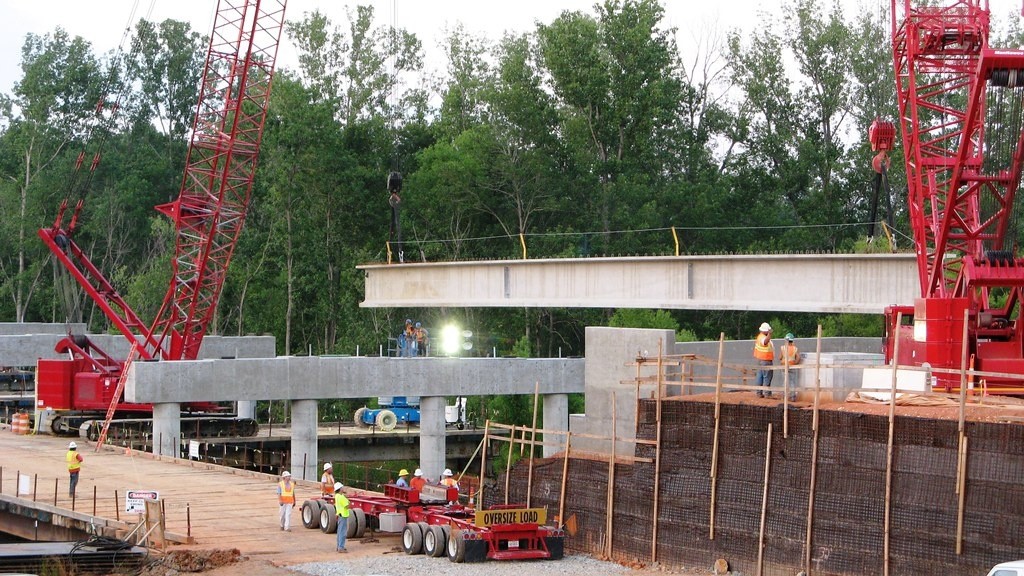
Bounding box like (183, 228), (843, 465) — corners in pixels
(286, 529), (291, 532)
(337, 549), (347, 552)
(69, 494), (78, 498)
(281, 526), (284, 530)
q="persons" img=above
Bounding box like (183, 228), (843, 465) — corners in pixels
(405, 319), (416, 357)
(780, 333), (804, 402)
(334, 482), (351, 554)
(395, 469), (410, 487)
(409, 469), (427, 492)
(416, 322), (428, 357)
(67, 442), (84, 498)
(276, 471), (296, 531)
(321, 463), (335, 494)
(438, 469), (460, 491)
(753, 322), (778, 399)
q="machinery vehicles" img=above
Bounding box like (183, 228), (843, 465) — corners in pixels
(866, 0), (1023, 400)
(32, 0), (290, 444)
(353, 322), (476, 433)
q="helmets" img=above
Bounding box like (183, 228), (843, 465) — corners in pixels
(68, 442), (77, 448)
(414, 469), (423, 476)
(443, 469), (453, 475)
(416, 322), (421, 328)
(324, 463), (332, 471)
(282, 471), (291, 477)
(334, 482), (343, 491)
(759, 322), (771, 332)
(407, 319), (411, 323)
(399, 469), (409, 476)
(785, 332), (794, 342)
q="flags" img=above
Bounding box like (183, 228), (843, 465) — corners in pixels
(566, 513), (577, 536)
(450, 518), (460, 544)
